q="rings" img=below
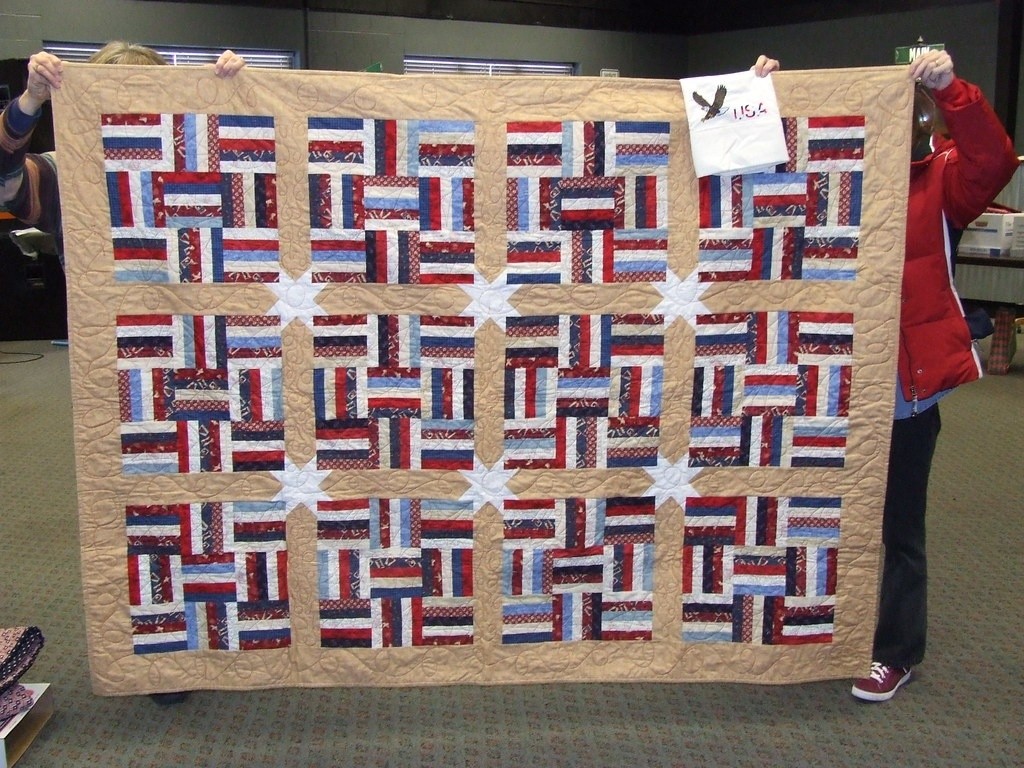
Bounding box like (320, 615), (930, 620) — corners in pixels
(933, 60), (939, 68)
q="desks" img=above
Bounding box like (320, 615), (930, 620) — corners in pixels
(954, 253), (1024, 305)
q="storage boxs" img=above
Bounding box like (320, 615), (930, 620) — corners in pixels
(968, 213), (1024, 257)
(0, 683), (53, 768)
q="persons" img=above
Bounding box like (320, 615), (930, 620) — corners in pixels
(0, 38), (248, 706)
(753, 48), (1020, 702)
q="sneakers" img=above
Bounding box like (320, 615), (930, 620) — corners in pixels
(851, 661), (913, 701)
